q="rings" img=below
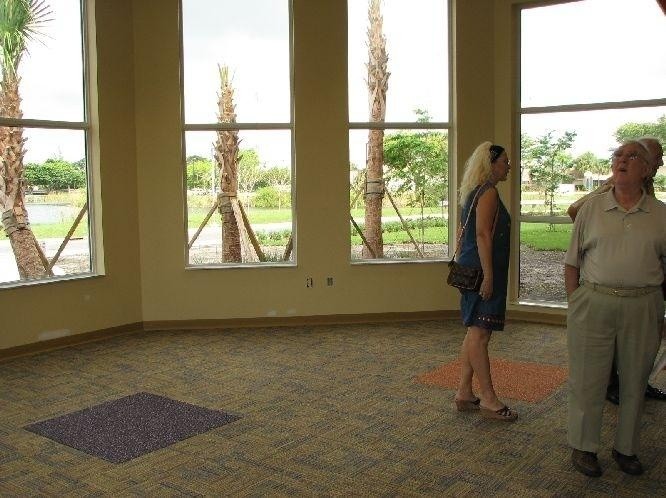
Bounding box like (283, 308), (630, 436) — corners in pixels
(479, 293), (483, 295)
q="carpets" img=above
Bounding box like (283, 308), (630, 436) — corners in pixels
(24, 389), (242, 466)
(410, 348), (569, 405)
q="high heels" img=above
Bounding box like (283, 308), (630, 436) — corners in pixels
(479, 401), (521, 421)
(454, 394), (486, 412)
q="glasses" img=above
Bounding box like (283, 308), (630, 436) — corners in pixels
(611, 150), (650, 167)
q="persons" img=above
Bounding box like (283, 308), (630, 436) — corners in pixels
(455, 141), (519, 421)
(568, 136), (666, 406)
(563, 138), (666, 478)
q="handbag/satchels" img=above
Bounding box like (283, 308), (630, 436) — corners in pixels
(446, 260), (484, 293)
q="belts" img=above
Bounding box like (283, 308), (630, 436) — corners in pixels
(573, 277), (662, 297)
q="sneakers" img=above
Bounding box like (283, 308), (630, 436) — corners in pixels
(611, 447), (646, 478)
(570, 446), (603, 478)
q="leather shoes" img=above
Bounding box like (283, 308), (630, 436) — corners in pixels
(606, 383), (621, 405)
(646, 383), (666, 404)
